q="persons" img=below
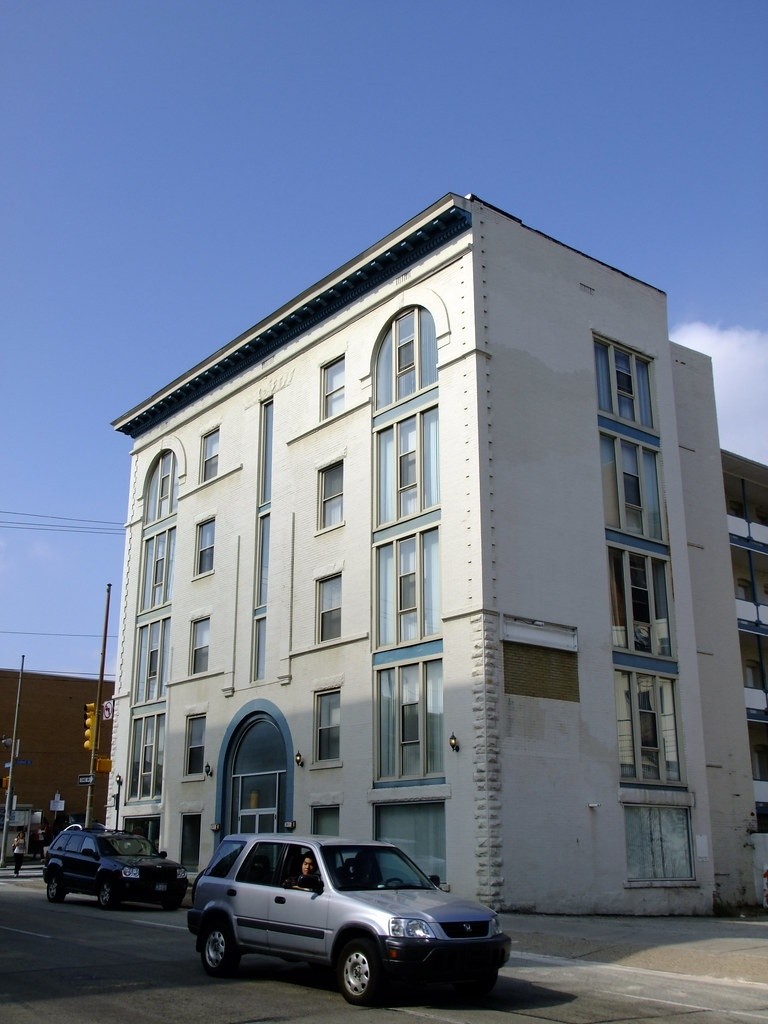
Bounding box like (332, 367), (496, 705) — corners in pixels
(13, 831), (27, 877)
(32, 822), (53, 861)
(352, 851), (382, 885)
(282, 850), (321, 892)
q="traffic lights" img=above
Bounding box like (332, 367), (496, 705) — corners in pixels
(82, 718), (95, 750)
(82, 702), (96, 729)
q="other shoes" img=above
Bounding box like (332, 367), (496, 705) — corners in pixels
(14, 873), (19, 878)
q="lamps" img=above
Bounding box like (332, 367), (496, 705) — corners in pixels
(204, 761), (213, 777)
(295, 749), (304, 768)
(116, 773), (123, 785)
(448, 731), (460, 752)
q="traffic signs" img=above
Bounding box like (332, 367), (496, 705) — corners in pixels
(78, 774), (95, 786)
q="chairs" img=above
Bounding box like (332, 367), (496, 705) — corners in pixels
(341, 857), (368, 882)
(250, 854), (274, 882)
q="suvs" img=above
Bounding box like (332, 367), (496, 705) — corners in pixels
(187, 833), (511, 1008)
(41, 823), (188, 912)
